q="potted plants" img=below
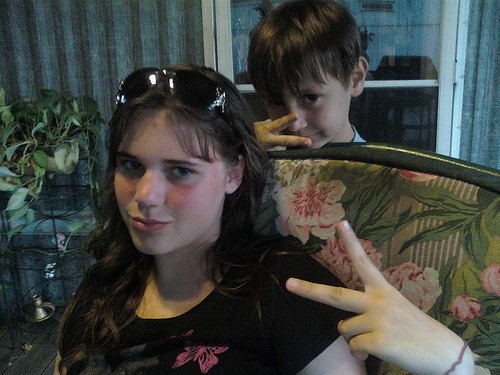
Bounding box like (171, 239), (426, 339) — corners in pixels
(0, 87), (106, 257)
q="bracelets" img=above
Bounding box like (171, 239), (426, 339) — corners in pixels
(444, 337), (469, 375)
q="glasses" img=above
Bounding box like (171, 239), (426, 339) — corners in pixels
(116, 67), (228, 117)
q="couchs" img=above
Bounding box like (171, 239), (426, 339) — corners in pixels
(267, 142), (500, 375)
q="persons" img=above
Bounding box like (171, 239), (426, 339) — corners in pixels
(56, 64), (493, 375)
(244, 1), (368, 146)
